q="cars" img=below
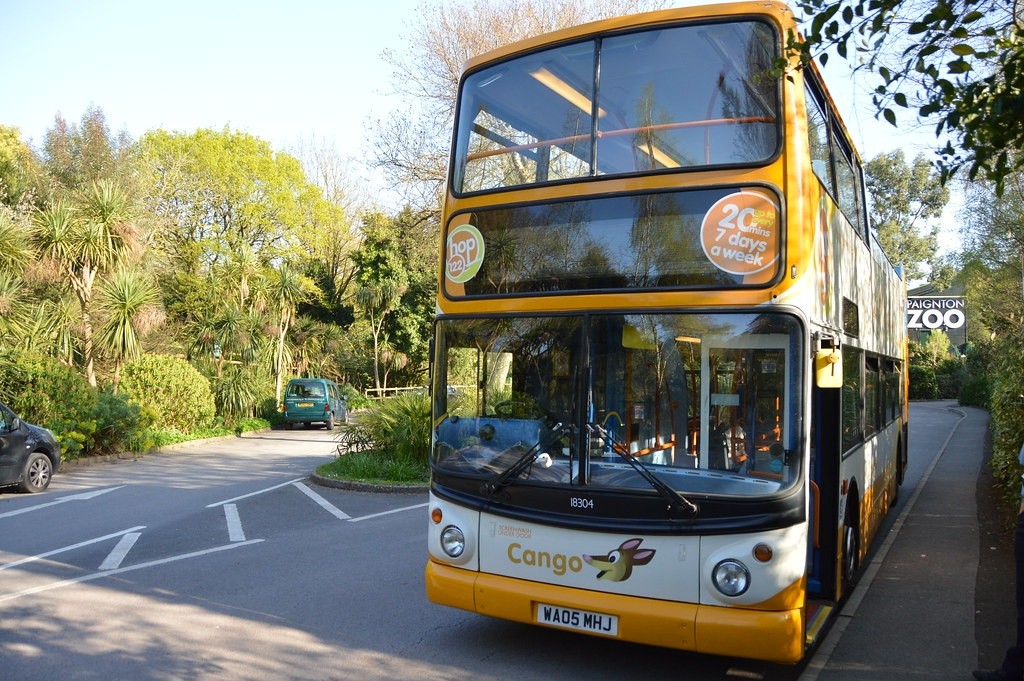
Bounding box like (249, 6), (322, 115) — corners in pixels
(1, 400), (63, 495)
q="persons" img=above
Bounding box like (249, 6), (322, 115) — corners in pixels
(719, 407), (748, 476)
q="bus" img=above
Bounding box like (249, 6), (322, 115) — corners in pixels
(421, 1), (911, 667)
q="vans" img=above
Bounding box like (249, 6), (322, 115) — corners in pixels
(282, 378), (350, 431)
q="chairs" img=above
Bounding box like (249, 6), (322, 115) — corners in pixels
(538, 372), (577, 432)
